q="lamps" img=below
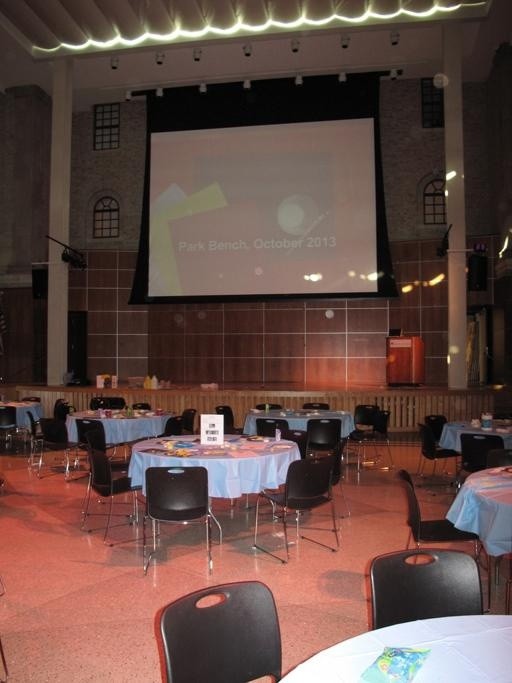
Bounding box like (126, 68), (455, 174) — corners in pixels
(44, 234), (88, 270)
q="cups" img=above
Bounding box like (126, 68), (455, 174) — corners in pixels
(97, 405), (164, 418)
(482, 412), (493, 431)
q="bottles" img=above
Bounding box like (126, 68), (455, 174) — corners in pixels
(264, 399), (270, 414)
(275, 422), (282, 441)
(143, 373), (172, 389)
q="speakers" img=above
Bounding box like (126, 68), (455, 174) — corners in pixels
(467, 253), (487, 292)
(32, 268), (48, 300)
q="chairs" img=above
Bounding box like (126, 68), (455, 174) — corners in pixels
(154, 580), (282, 683)
(398, 468), (481, 566)
(416, 405), (512, 490)
(364, 546), (492, 632)
